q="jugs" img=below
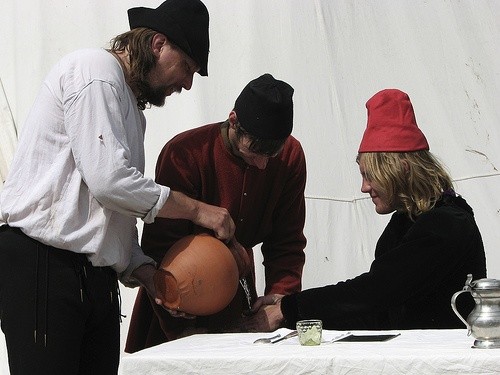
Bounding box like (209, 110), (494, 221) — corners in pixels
(154, 229), (251, 316)
(450, 273), (500, 349)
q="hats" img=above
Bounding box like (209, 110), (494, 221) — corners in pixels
(358, 88), (429, 152)
(127, 0), (209, 76)
(234, 74), (295, 137)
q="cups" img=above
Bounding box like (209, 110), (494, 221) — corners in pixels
(296, 320), (323, 346)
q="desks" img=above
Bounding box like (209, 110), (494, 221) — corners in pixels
(118, 328), (499, 375)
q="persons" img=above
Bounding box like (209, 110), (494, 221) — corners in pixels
(0, 0), (211, 374)
(124, 72), (308, 353)
(236, 89), (488, 333)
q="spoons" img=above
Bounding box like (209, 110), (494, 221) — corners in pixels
(253, 334), (282, 344)
